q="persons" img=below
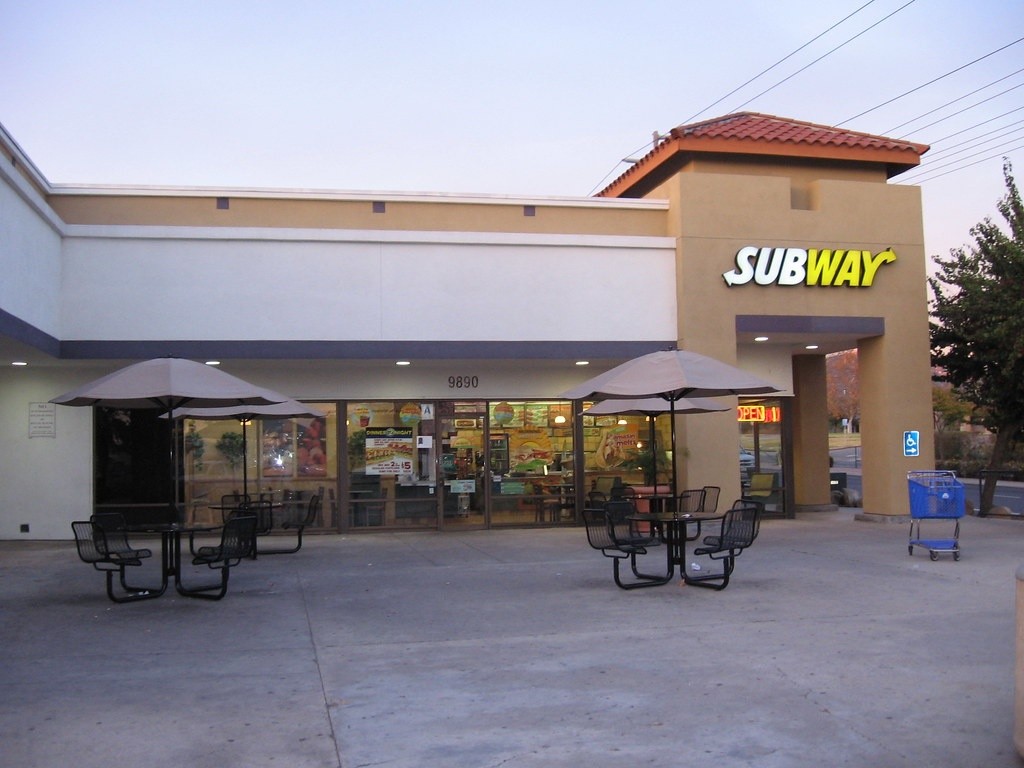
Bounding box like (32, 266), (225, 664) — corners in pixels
(641, 442), (669, 486)
(549, 455), (562, 471)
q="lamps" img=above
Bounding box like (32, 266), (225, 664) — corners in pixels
(555, 399), (566, 424)
(618, 415), (627, 424)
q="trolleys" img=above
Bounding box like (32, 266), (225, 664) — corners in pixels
(906, 470), (966, 561)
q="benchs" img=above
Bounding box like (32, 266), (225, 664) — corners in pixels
(745, 473), (779, 513)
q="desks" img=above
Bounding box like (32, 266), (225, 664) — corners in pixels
(209, 501), (284, 559)
(622, 494), (690, 538)
(112, 523), (225, 596)
(245, 491), (282, 527)
(626, 512), (727, 586)
(347, 491), (374, 527)
(542, 483), (593, 522)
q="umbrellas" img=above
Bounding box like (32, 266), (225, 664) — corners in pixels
(48, 353), (289, 505)
(556, 346), (787, 519)
(157, 387), (328, 502)
(576, 397), (732, 495)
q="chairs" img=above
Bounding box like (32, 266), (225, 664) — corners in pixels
(363, 488), (388, 527)
(531, 479), (765, 593)
(328, 487), (355, 528)
(70, 486), (326, 605)
